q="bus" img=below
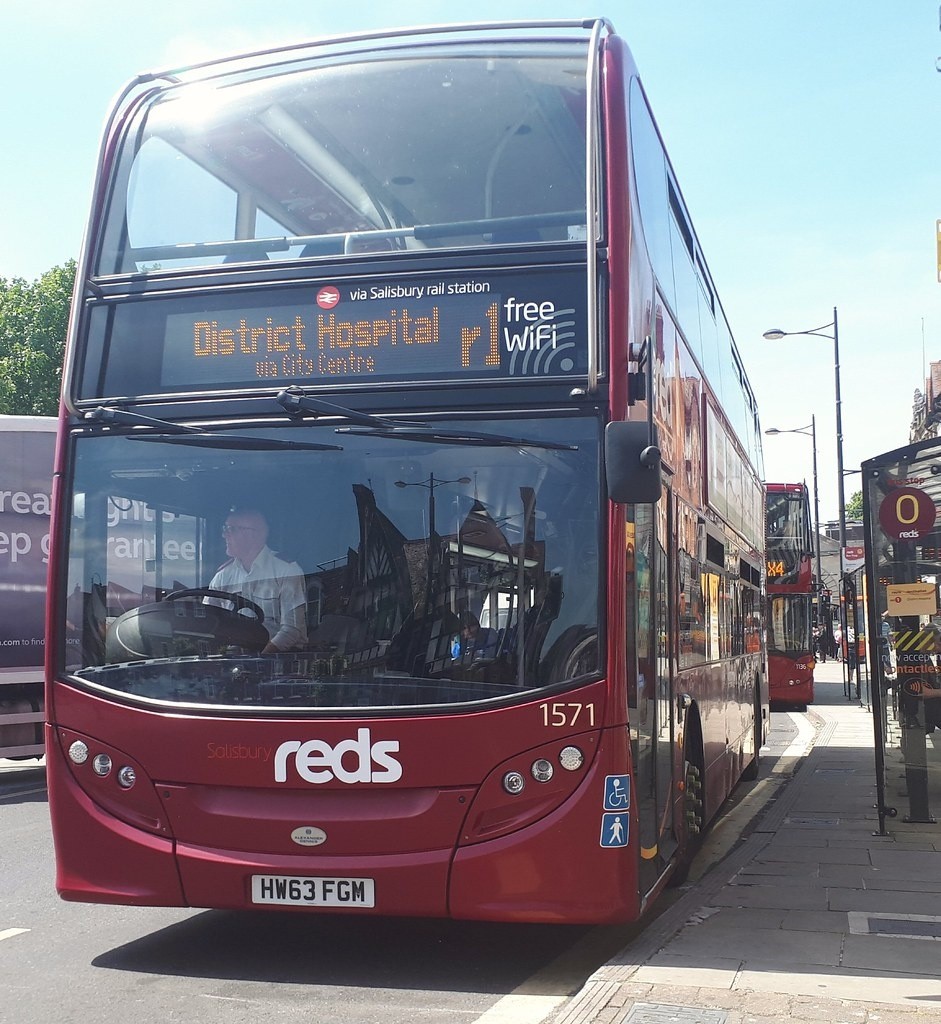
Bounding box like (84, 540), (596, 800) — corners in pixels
(762, 482), (820, 712)
(37, 16), (772, 932)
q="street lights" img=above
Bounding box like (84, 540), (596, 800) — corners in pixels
(764, 414), (826, 665)
(761, 308), (853, 703)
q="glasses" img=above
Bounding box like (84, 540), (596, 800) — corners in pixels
(222, 524), (257, 533)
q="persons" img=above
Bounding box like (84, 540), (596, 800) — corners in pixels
(203, 507), (310, 658)
(881, 615), (941, 733)
(811, 621), (841, 664)
(451, 611), (503, 680)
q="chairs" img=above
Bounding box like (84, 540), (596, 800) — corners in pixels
(491, 228), (545, 243)
(223, 253), (269, 263)
(299, 242), (342, 256)
